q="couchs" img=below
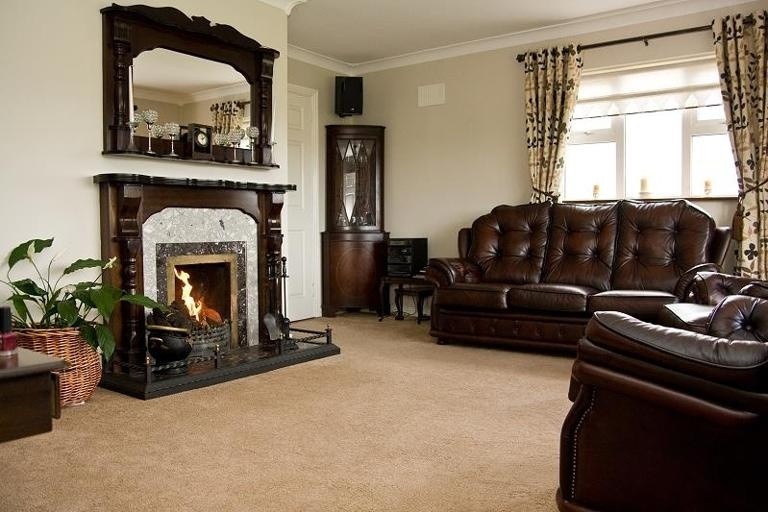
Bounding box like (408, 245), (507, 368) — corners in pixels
(431, 200), (735, 354)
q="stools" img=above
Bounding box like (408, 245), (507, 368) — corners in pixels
(395, 286), (432, 325)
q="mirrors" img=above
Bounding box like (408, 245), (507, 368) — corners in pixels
(98, 2), (281, 165)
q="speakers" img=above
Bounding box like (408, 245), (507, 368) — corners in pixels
(334, 75), (363, 116)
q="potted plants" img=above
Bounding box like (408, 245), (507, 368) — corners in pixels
(0, 234), (165, 409)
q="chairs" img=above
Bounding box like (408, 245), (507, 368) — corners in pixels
(564, 261), (766, 512)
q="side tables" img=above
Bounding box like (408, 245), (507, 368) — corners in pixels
(378, 276), (433, 321)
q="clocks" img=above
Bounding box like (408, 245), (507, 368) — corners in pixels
(188, 121), (213, 160)
